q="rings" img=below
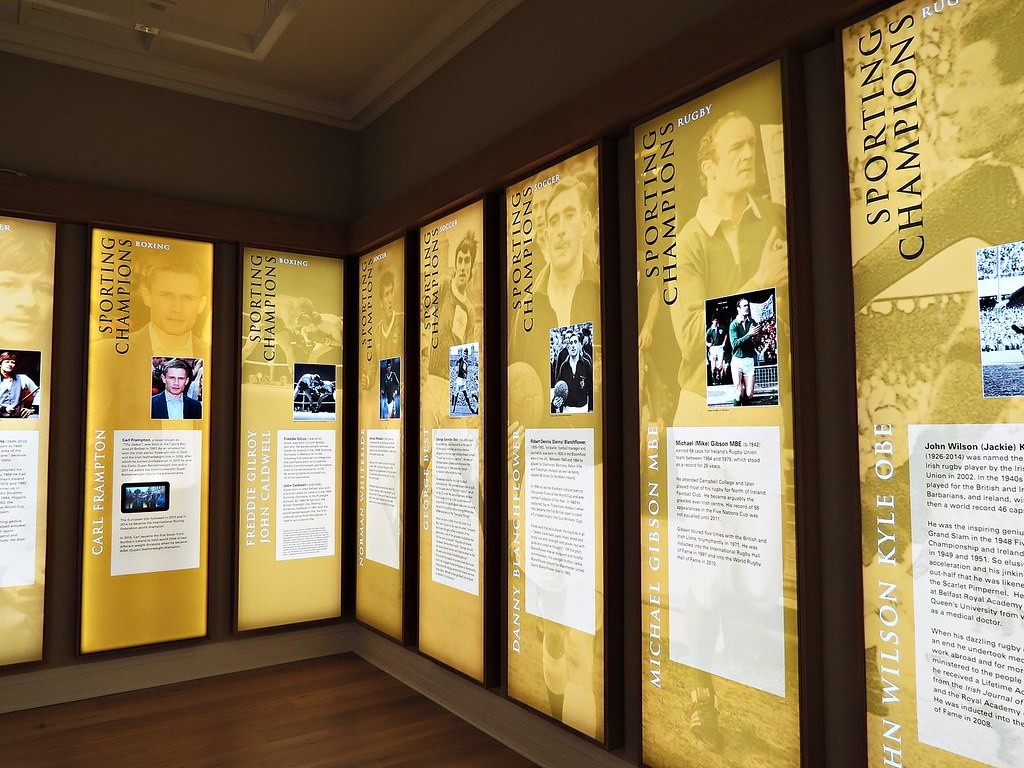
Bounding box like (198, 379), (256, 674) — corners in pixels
(776, 243), (782, 249)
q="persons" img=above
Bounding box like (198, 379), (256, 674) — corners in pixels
(509, 162), (600, 484)
(980, 306), (1024, 358)
(152, 359), (202, 419)
(941, 0), (1024, 171)
(0, 352), (40, 418)
(132, 493), (162, 508)
(639, 350), (664, 455)
(420, 231), (479, 495)
(312, 380), (335, 413)
(361, 271), (399, 418)
(294, 374), (321, 411)
(242, 294), (313, 373)
(152, 357), (203, 401)
(667, 112), (789, 427)
(637, 258), (660, 407)
(705, 298), (777, 405)
(302, 312), (343, 363)
(94, 253), (209, 376)
(0, 220), (54, 344)
